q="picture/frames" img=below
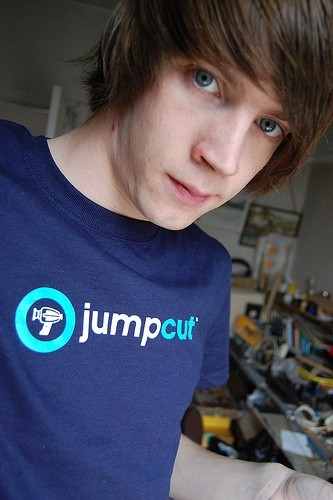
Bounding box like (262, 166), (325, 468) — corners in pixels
(239, 203), (303, 248)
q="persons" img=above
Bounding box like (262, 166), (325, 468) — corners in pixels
(0, 0), (333, 500)
